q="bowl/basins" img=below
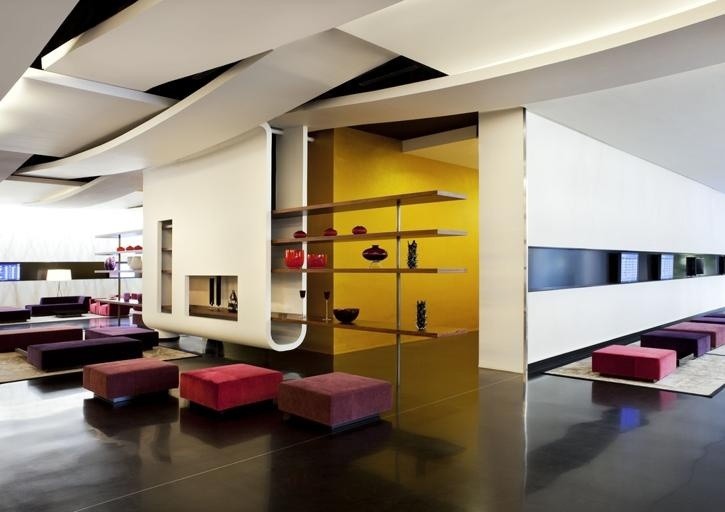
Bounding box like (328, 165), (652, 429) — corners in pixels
(126, 255), (142, 270)
(332, 309), (359, 323)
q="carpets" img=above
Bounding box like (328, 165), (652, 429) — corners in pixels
(542, 339), (724, 398)
(0, 330), (201, 386)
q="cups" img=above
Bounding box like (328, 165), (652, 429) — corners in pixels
(285, 249), (304, 269)
(123, 292), (129, 302)
(307, 254), (327, 269)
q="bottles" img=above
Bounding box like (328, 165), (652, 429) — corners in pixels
(228, 290), (238, 312)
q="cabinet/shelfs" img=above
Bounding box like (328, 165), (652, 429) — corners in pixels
(94, 229), (142, 307)
(271, 189), (472, 340)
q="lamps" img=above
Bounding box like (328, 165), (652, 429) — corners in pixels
(46, 269), (72, 296)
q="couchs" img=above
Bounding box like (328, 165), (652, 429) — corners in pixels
(0, 324), (84, 354)
(278, 371), (393, 434)
(24, 296), (91, 317)
(180, 362), (282, 414)
(590, 307), (724, 383)
(27, 337), (143, 374)
(86, 325), (159, 350)
(0, 306), (31, 323)
(82, 357), (180, 404)
